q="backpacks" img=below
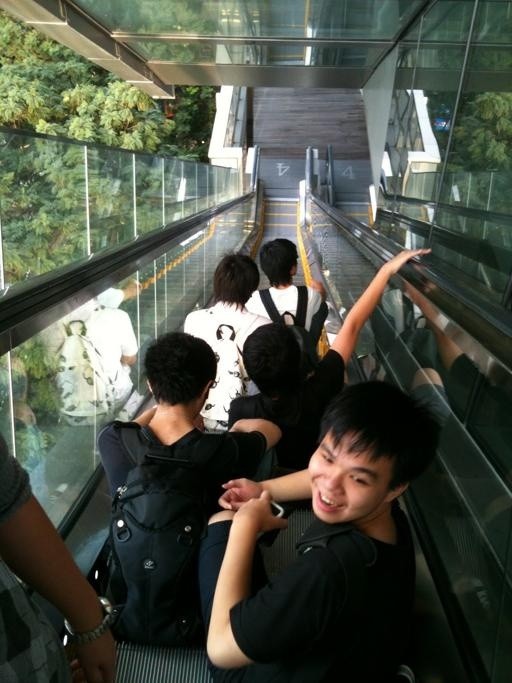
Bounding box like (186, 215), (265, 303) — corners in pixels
(260, 286), (318, 379)
(200, 312), (257, 429)
(55, 307), (115, 428)
(99, 422), (222, 649)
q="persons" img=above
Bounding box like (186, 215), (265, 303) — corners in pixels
(413, 329), (493, 431)
(0, 418), (125, 683)
(242, 236), (329, 354)
(96, 332), (283, 650)
(195, 378), (448, 682)
(38, 287), (139, 427)
(183, 254), (274, 434)
(0, 356), (46, 504)
(228, 246), (434, 469)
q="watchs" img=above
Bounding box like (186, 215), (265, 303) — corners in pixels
(63, 595), (119, 644)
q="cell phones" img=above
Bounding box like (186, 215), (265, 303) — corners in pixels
(255, 499), (284, 540)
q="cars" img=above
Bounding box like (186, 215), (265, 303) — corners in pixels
(429, 100), (451, 133)
